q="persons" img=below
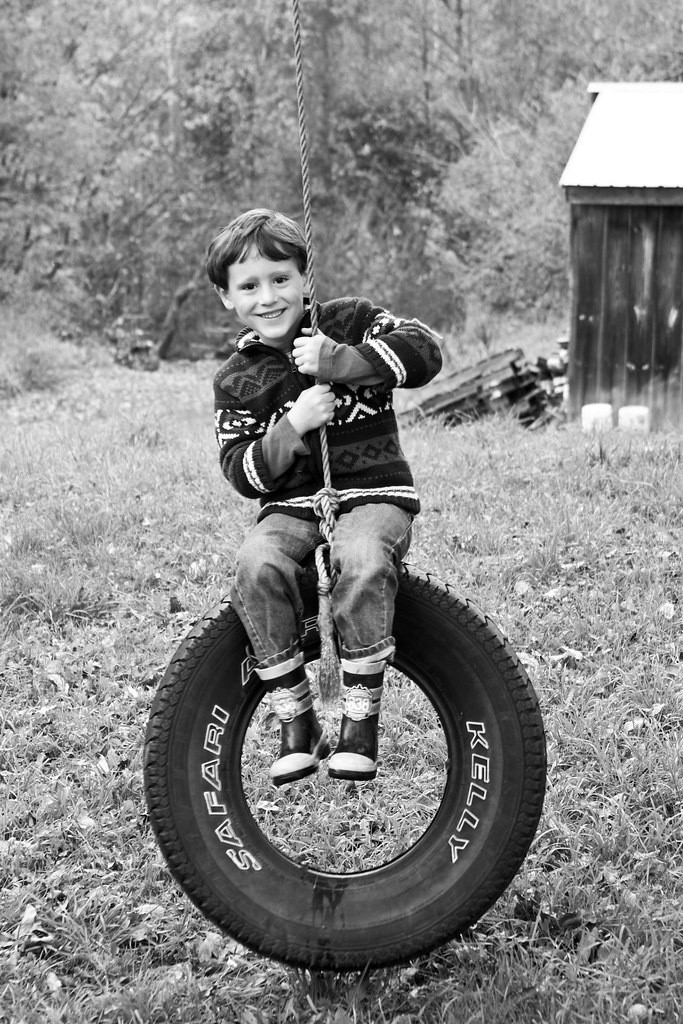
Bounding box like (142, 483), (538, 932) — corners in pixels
(207, 208), (443, 787)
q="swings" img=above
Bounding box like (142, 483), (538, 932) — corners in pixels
(143, 3), (549, 971)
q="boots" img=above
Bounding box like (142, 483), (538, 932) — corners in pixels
(252, 651), (330, 786)
(328, 658), (386, 781)
